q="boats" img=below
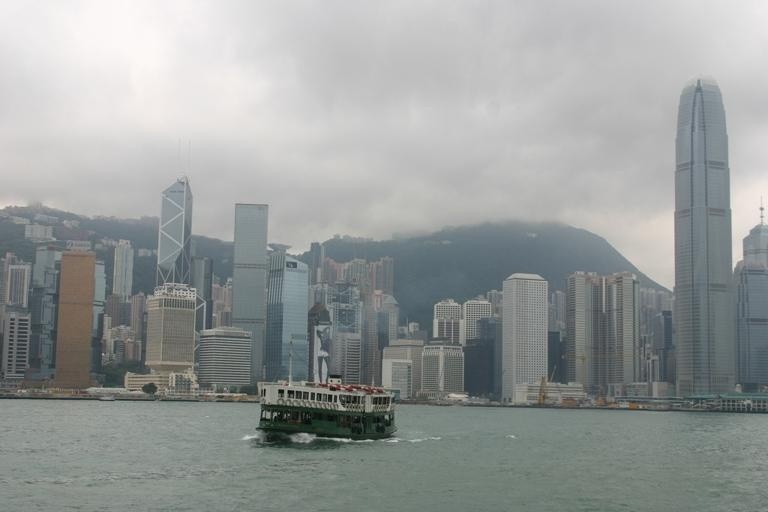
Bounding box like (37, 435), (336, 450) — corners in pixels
(255, 340), (398, 440)
